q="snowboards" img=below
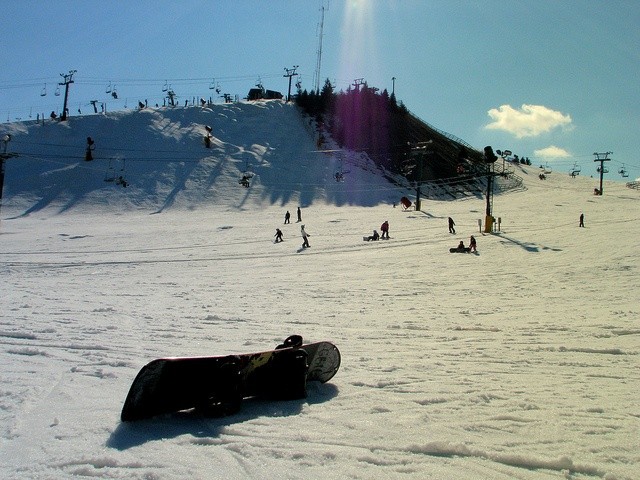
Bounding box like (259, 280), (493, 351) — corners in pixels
(449, 248), (471, 253)
(379, 237), (390, 239)
(363, 237), (370, 241)
(121, 341), (341, 422)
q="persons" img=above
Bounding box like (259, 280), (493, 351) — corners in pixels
(296, 207), (303, 224)
(456, 241), (465, 254)
(448, 217), (456, 234)
(469, 235), (478, 254)
(579, 214), (585, 228)
(284, 211), (291, 225)
(300, 225), (312, 249)
(274, 228), (283, 243)
(380, 222), (390, 239)
(365, 231), (378, 242)
